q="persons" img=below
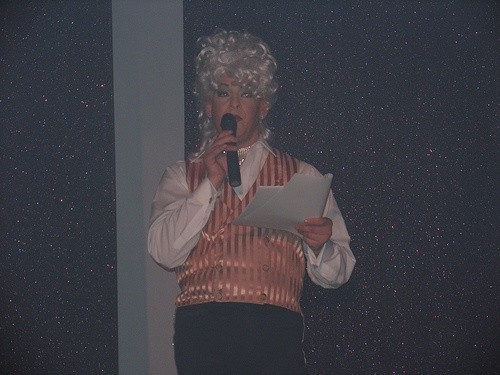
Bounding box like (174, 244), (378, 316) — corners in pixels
(146, 29), (356, 375)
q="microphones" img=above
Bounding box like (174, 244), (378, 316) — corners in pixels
(222, 113), (242, 187)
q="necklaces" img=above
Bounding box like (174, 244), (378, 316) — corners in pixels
(222, 145), (253, 166)
